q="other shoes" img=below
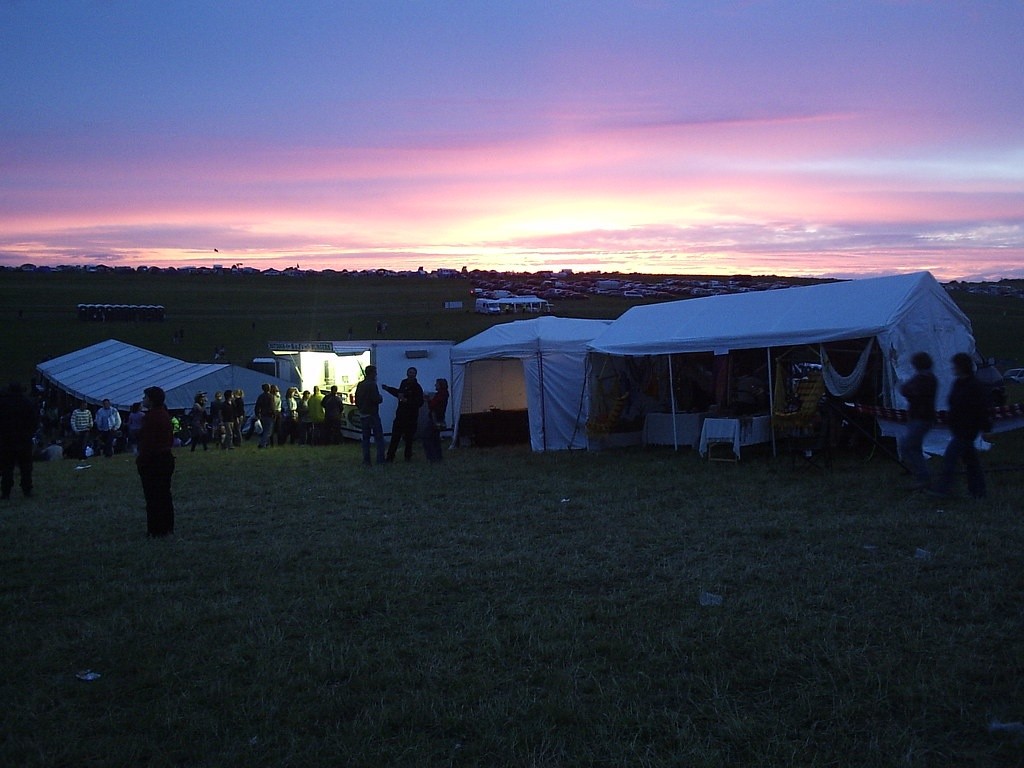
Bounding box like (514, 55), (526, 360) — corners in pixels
(910, 481), (930, 490)
(963, 492), (986, 500)
(24, 489), (38, 496)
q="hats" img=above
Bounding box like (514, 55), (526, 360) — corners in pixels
(197, 391), (208, 395)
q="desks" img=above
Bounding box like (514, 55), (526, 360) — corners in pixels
(641, 413), (720, 448)
(699, 416), (771, 463)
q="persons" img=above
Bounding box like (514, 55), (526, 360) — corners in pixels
(376, 321), (388, 334)
(505, 304), (526, 313)
(348, 327), (353, 340)
(135, 386), (175, 539)
(172, 325), (185, 345)
(213, 343), (226, 362)
(39, 365), (449, 465)
(0, 382), (42, 500)
(896, 351), (992, 496)
(731, 363), (770, 464)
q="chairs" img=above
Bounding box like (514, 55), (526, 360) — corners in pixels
(788, 408), (833, 476)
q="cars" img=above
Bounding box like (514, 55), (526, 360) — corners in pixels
(1002, 368), (1024, 382)
(430, 268), (804, 314)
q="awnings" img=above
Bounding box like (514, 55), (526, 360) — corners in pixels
(268, 340), (372, 356)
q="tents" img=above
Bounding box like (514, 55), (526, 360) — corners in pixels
(584, 271), (978, 454)
(449, 316), (618, 454)
(493, 296), (549, 314)
(33, 336), (301, 435)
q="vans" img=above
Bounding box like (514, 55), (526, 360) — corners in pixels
(475, 298), (500, 316)
